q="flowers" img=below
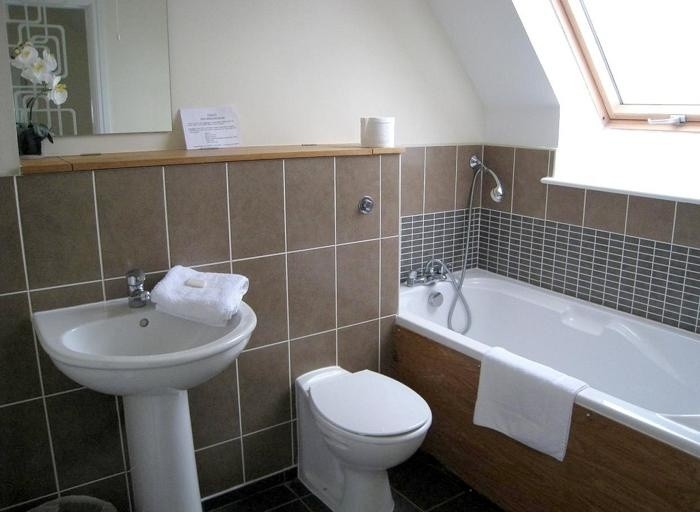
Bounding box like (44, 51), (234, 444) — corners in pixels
(12, 42), (69, 128)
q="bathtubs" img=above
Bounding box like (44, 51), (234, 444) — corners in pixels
(394, 267), (700, 459)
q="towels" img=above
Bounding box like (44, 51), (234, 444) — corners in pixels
(473, 342), (590, 463)
(150, 264), (249, 327)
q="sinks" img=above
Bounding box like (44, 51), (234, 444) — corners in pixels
(32, 296), (258, 396)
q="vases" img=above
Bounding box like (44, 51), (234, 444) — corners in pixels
(19, 126), (44, 154)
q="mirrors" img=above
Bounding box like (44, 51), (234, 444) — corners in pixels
(89, 1), (174, 136)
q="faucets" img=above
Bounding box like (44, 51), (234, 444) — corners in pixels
(125, 269), (151, 308)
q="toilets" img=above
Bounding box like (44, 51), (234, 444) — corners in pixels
(295, 364), (433, 512)
